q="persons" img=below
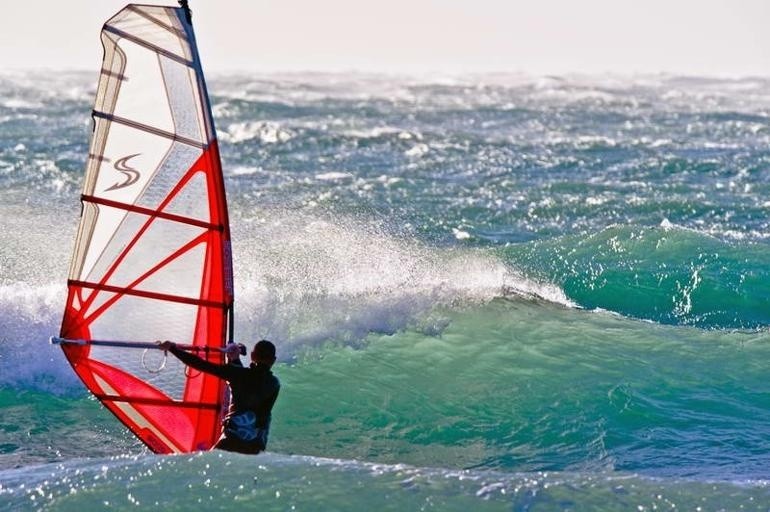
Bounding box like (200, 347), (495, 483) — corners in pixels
(161, 340), (280, 454)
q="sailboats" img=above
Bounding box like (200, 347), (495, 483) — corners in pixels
(48, 0), (236, 455)
